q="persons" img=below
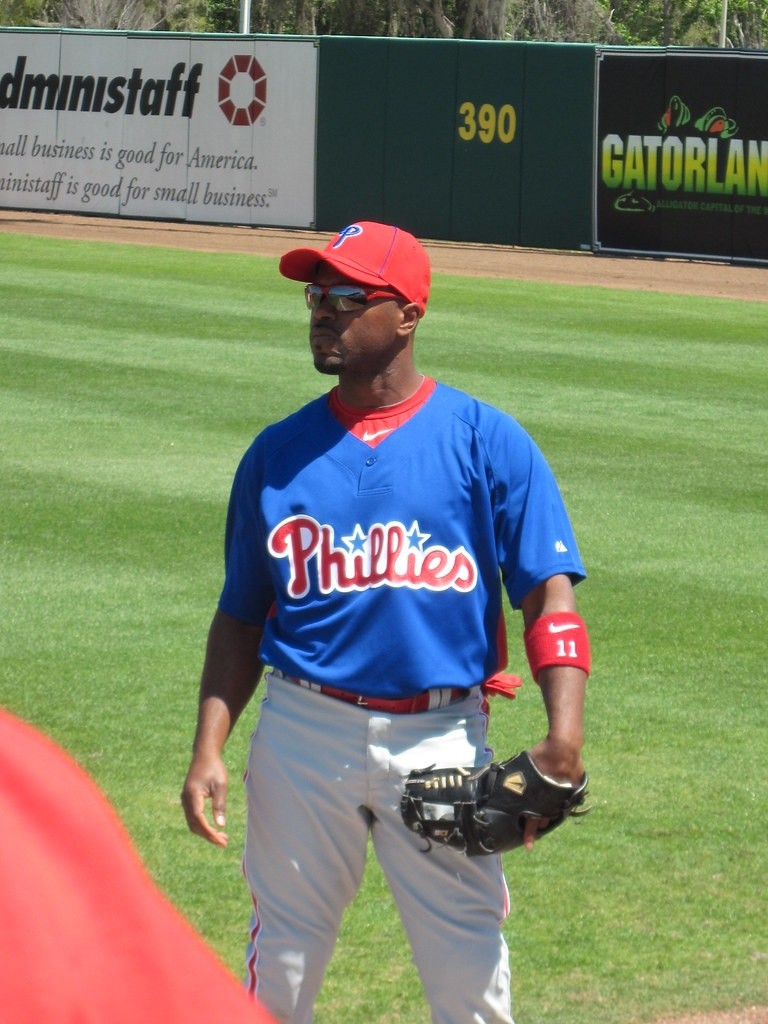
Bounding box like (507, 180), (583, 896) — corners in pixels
(183, 220), (585, 1024)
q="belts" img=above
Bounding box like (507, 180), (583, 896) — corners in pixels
(271, 665), (473, 713)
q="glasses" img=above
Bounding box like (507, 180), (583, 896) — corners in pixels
(306, 284), (401, 312)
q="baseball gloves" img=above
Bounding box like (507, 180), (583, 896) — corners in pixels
(398, 749), (590, 858)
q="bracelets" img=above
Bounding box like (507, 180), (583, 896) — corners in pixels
(522, 611), (589, 682)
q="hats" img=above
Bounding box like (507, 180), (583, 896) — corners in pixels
(280, 221), (431, 315)
